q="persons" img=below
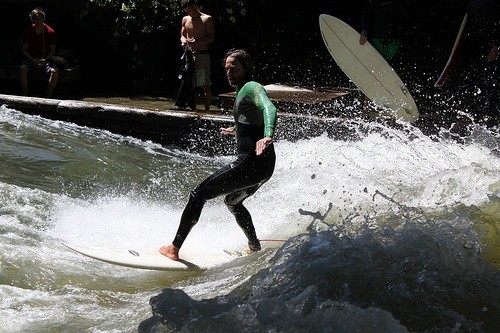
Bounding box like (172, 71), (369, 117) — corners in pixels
(18, 8), (59, 98)
(171, 0), (214, 113)
(160, 48), (277, 261)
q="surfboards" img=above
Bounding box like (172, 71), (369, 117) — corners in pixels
(319, 14), (420, 123)
(62, 241), (271, 272)
